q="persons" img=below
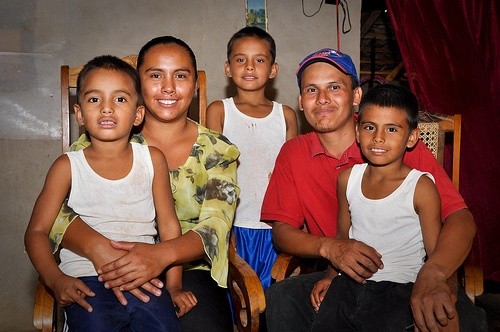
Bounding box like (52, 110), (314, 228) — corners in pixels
(48, 36), (241, 332)
(308, 83), (443, 332)
(24, 55), (199, 332)
(206, 27), (298, 292)
(260, 48), (482, 332)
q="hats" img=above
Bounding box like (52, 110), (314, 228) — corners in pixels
(295, 48), (359, 88)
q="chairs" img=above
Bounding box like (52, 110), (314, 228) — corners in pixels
(32, 54), (266, 332)
(269, 113), (484, 305)
